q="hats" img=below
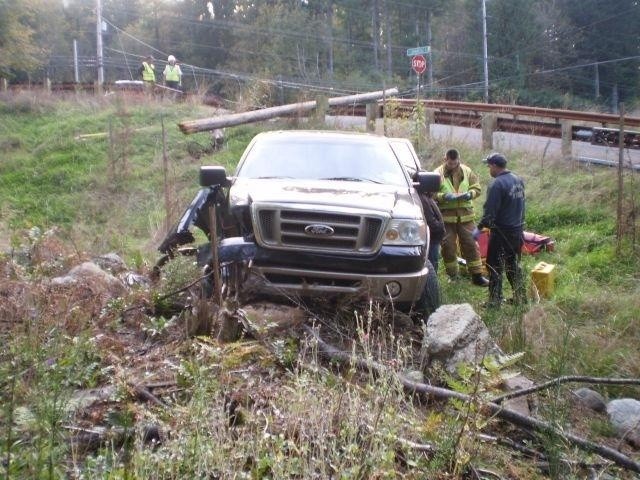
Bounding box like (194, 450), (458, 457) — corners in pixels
(482, 153), (505, 167)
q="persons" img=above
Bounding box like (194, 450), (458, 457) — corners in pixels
(138, 56), (157, 94)
(429, 149), (490, 286)
(162, 55), (183, 89)
(413, 170), (446, 274)
(472, 153), (527, 309)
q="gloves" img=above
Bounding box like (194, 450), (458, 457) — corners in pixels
(445, 192), (471, 201)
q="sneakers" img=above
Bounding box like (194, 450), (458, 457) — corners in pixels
(471, 275), (490, 287)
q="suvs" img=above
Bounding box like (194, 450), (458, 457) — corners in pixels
(200, 130), (441, 318)
(159, 136), (446, 274)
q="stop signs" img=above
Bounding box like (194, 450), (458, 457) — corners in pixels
(412, 55), (426, 74)
